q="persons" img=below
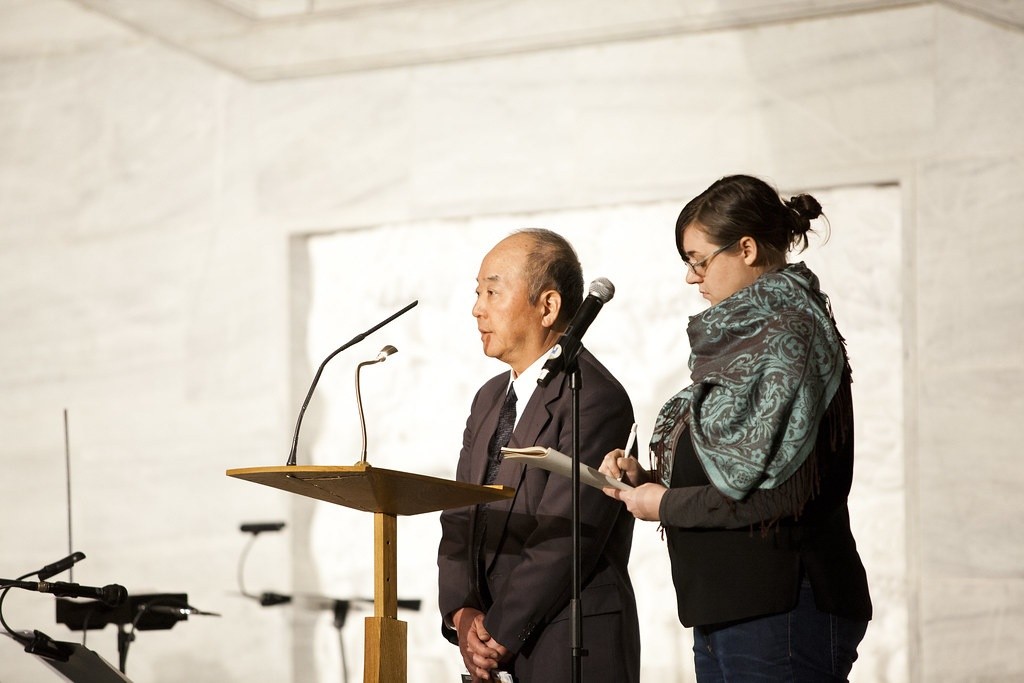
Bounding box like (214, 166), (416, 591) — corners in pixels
(598, 174), (873, 683)
(435, 230), (640, 683)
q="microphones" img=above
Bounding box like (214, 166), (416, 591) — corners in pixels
(286, 300), (418, 466)
(21, 582), (128, 607)
(537, 278), (615, 388)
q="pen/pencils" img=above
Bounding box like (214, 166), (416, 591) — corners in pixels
(616, 423), (638, 482)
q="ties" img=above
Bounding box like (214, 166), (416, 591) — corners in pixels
(471, 384), (517, 617)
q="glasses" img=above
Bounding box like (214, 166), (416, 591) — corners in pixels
(685, 237), (741, 274)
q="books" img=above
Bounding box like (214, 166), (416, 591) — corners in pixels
(461, 672), (513, 683)
(501, 446), (634, 492)
